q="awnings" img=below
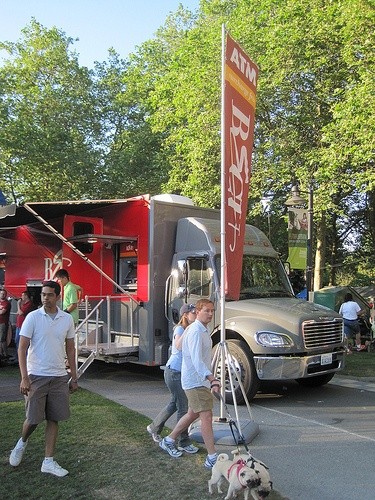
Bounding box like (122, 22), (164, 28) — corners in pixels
(0, 198), (142, 307)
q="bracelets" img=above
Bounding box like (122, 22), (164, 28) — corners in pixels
(210, 378), (220, 389)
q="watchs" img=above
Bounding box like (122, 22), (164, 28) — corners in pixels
(70, 377), (79, 385)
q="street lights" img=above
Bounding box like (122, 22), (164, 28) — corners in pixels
(284, 186), (312, 292)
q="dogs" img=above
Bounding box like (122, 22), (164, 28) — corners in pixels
(207, 446), (274, 500)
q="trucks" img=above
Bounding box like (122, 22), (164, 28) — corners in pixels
(0, 194), (348, 405)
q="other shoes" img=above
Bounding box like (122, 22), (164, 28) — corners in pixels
(0, 355), (7, 361)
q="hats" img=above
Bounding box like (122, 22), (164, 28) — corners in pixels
(180, 304), (196, 315)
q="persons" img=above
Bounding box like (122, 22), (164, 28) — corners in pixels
(0, 288), (11, 366)
(55, 268), (83, 382)
(159, 298), (228, 468)
(147, 303), (200, 454)
(14, 289), (37, 369)
(9, 280), (78, 477)
(339, 293), (366, 351)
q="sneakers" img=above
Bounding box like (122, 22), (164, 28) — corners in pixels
(159, 438), (183, 457)
(345, 350), (352, 355)
(357, 345), (365, 351)
(147, 425), (165, 442)
(178, 444), (199, 453)
(9, 437), (28, 466)
(204, 454), (219, 468)
(41, 461), (69, 477)
